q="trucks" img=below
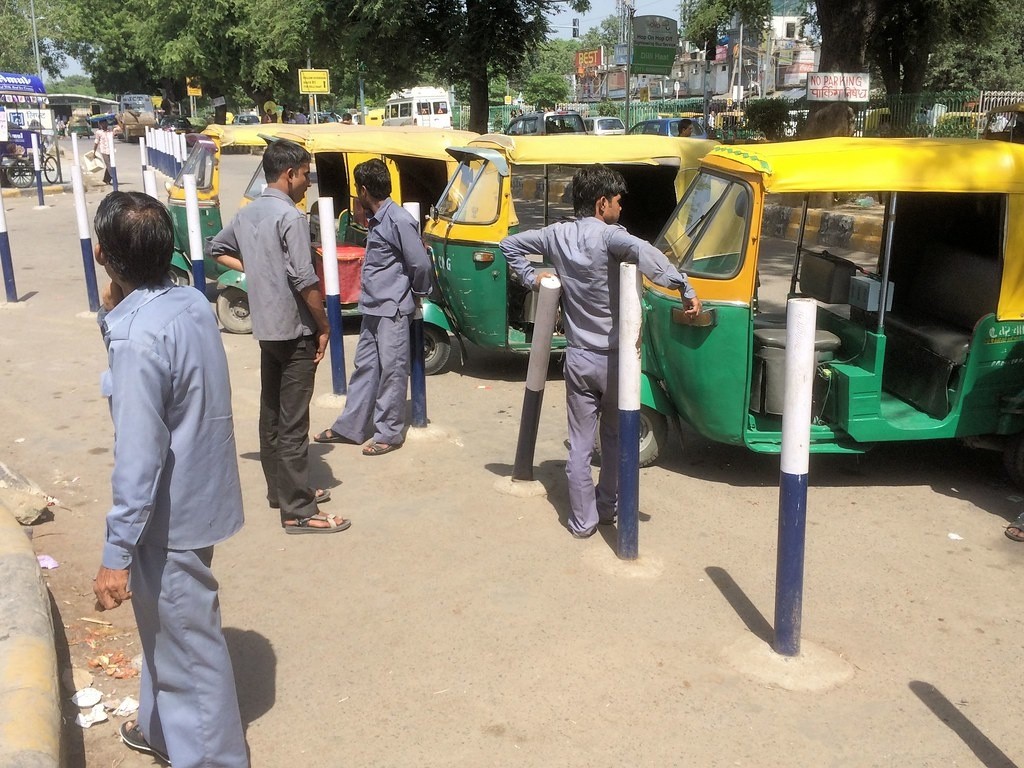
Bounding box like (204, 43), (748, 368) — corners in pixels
(119, 94), (158, 142)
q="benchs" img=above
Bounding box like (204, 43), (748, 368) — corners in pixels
(850, 223), (1004, 417)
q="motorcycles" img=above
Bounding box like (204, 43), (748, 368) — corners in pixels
(398, 133), (762, 390)
(214, 127), (521, 335)
(590, 135), (1024, 493)
(659, 96), (1024, 144)
(163, 121), (407, 296)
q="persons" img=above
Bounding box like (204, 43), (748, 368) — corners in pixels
(499, 162), (703, 539)
(261, 107), (354, 125)
(508, 106), (565, 134)
(312, 158), (433, 457)
(204, 137), (354, 536)
(676, 119), (692, 138)
(93, 120), (114, 183)
(86, 190), (258, 768)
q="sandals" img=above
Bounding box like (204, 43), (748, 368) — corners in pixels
(120, 719), (173, 766)
(283, 512), (352, 534)
(267, 487), (331, 508)
(313, 429), (361, 445)
(363, 440), (402, 455)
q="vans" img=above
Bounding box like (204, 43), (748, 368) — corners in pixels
(382, 88), (453, 130)
(503, 110), (589, 138)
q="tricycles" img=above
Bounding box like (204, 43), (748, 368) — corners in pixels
(3, 148), (60, 185)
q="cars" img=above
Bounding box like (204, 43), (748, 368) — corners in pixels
(584, 117), (626, 136)
(230, 113), (261, 126)
(625, 118), (707, 138)
(281, 110), (362, 127)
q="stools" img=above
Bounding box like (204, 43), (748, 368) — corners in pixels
(750, 313), (840, 415)
(309, 240), (367, 304)
